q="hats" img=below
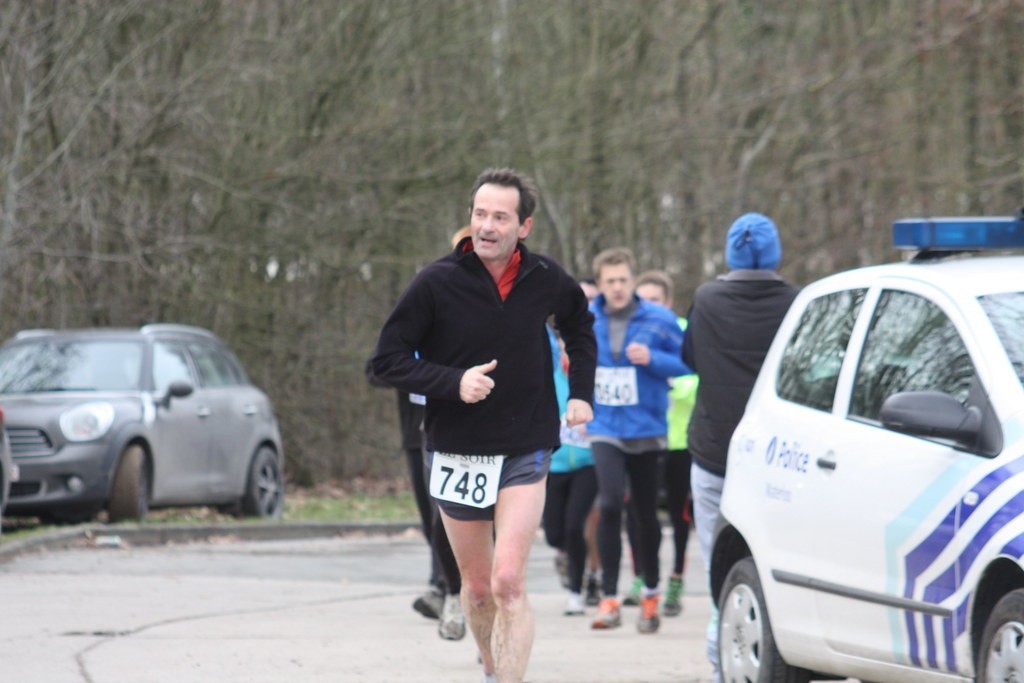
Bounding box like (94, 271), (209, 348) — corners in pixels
(724, 213), (781, 269)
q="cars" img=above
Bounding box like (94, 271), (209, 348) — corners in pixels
(706, 210), (1024, 683)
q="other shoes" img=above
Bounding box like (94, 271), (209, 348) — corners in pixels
(586, 579), (600, 604)
(566, 595), (582, 616)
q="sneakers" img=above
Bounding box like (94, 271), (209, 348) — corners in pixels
(624, 581), (641, 604)
(639, 595), (658, 631)
(591, 601), (619, 628)
(415, 593), (444, 618)
(665, 580), (682, 614)
(439, 615), (465, 639)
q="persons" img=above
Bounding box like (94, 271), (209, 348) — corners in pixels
(363, 165), (800, 683)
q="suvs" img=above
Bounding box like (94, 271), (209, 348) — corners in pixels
(0, 317), (286, 531)
